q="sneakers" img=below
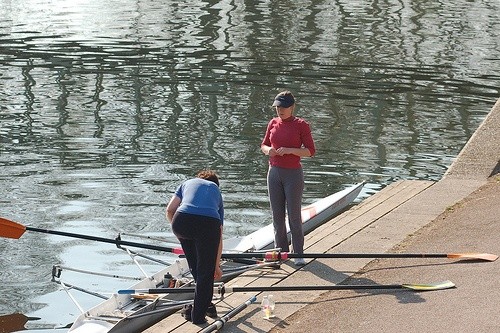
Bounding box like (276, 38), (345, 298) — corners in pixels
(181, 303), (192, 321)
(205, 302), (218, 318)
(294, 257), (306, 266)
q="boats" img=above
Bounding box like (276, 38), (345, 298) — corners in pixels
(67, 179), (368, 333)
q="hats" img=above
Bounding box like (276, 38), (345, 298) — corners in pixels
(270, 91), (294, 108)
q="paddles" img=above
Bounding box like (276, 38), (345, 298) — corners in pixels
(174, 250), (500, 264)
(196, 296), (257, 333)
(118, 279), (458, 294)
(0, 218), (264, 273)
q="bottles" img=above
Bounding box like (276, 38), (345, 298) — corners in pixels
(163, 271), (172, 288)
(260, 295), (269, 319)
(268, 295), (275, 317)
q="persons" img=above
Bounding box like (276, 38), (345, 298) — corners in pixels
(260, 91), (316, 264)
(166, 170), (224, 324)
